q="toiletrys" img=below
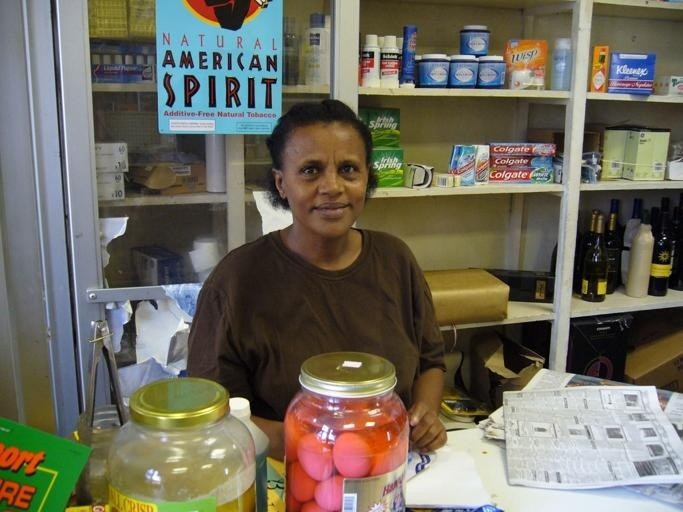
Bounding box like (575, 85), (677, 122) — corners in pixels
(281, 12), (331, 90)
(361, 33), (403, 91)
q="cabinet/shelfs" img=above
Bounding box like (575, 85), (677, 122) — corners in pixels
(57, 0), (359, 411)
(352, 0), (683, 390)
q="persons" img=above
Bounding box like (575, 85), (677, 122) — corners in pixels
(187, 98), (448, 462)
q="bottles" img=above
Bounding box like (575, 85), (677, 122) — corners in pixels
(622, 197), (643, 287)
(478, 56), (506, 89)
(397, 37), (403, 51)
(668, 206), (683, 290)
(574, 209), (600, 295)
(282, 351), (409, 512)
(660, 196), (671, 212)
(625, 211), (655, 298)
(282, 15), (301, 85)
(417, 53), (450, 89)
(648, 211), (673, 296)
(378, 37), (385, 48)
(70, 405), (131, 512)
(227, 397), (269, 512)
(300, 13), (331, 86)
(605, 198), (621, 294)
(109, 375), (258, 512)
(459, 25), (491, 56)
(648, 206), (660, 233)
(450, 54), (478, 88)
(549, 37), (572, 91)
(361, 35), (380, 88)
(380, 35), (400, 89)
(581, 212), (609, 302)
